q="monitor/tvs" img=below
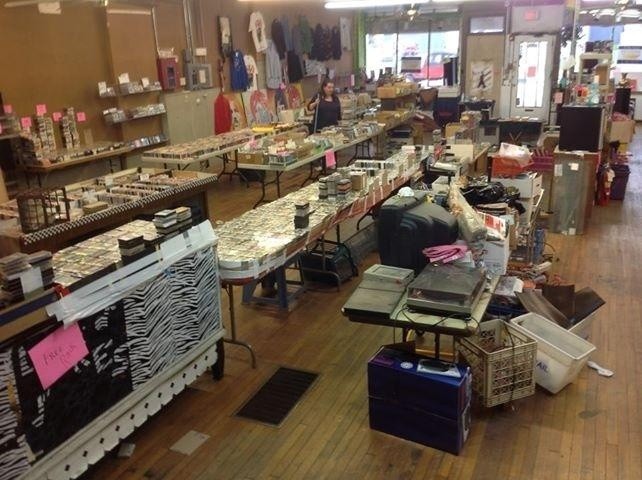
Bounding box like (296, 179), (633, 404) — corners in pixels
(379, 189), (459, 277)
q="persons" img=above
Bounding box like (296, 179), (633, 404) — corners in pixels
(307, 78), (341, 172)
(476, 72), (487, 90)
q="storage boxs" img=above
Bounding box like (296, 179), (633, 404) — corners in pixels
(364, 341), (477, 457)
(509, 311), (598, 397)
(433, 92), (634, 238)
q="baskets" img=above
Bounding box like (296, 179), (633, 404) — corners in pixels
(491, 150), (554, 179)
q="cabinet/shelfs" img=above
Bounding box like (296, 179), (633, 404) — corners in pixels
(2, 208), (225, 475)
(577, 54), (611, 110)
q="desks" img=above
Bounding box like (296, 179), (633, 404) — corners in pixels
(341, 187), (546, 359)
(0, 92), (415, 251)
(212, 142), (453, 372)
